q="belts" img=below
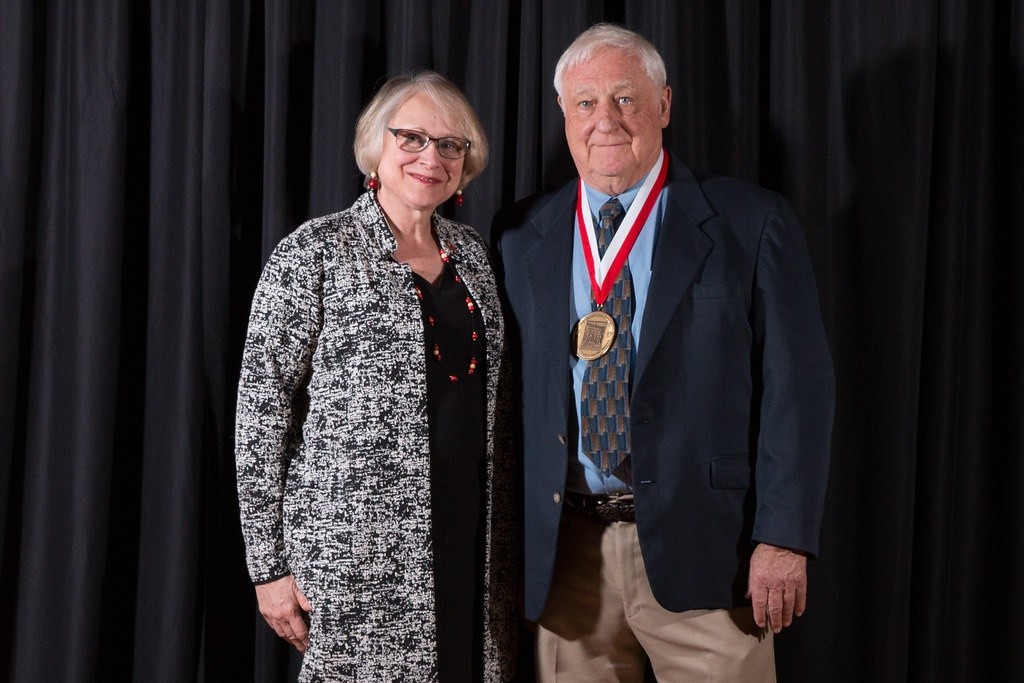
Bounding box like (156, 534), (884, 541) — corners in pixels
(562, 489), (636, 521)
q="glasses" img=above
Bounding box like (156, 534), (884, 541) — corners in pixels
(386, 127), (472, 159)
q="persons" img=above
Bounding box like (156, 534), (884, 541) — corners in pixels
(234, 70), (519, 683)
(489, 23), (835, 683)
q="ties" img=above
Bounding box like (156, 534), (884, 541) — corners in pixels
(581, 191), (634, 479)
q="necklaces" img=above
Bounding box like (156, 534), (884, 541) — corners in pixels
(416, 248), (478, 381)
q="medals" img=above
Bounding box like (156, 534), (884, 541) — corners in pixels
(571, 311), (615, 360)
